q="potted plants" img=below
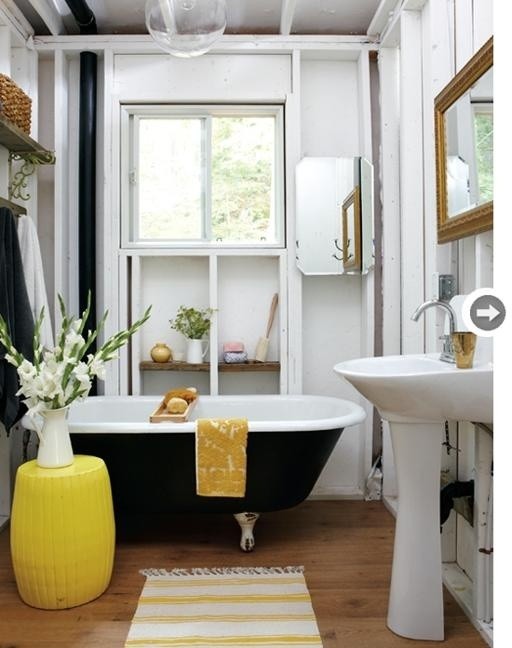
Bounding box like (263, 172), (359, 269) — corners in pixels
(167, 302), (216, 366)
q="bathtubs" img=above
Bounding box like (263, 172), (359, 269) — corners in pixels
(20, 394), (366, 514)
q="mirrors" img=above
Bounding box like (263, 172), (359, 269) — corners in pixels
(339, 184), (364, 273)
(431, 34), (493, 247)
(293, 151), (376, 278)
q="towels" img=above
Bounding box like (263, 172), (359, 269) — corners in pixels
(192, 417), (252, 498)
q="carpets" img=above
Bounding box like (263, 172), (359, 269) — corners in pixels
(122, 564), (325, 648)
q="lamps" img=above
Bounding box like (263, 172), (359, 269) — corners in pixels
(142, 0), (229, 59)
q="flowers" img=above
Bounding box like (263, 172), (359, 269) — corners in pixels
(0, 287), (157, 410)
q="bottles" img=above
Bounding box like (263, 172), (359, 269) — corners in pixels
(151, 343), (171, 362)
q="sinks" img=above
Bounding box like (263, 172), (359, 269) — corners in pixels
(331, 352), (493, 425)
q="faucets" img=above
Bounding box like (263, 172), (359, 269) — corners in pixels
(410, 299), (459, 365)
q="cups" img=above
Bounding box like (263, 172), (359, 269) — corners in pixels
(451, 331), (477, 368)
(186, 338), (210, 365)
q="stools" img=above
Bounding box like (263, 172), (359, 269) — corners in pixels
(9, 455), (117, 612)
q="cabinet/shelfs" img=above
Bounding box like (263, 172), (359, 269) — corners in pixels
(129, 248), (289, 396)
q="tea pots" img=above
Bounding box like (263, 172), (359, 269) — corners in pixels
(31, 402), (76, 469)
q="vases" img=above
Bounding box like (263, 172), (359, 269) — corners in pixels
(37, 403), (77, 472)
